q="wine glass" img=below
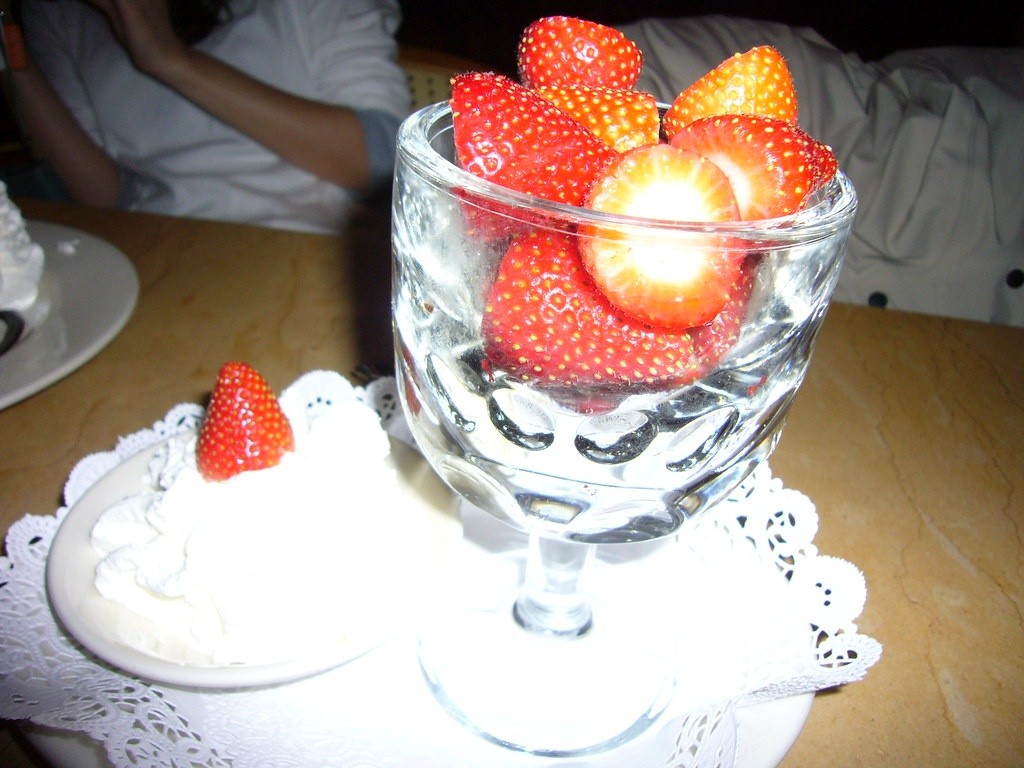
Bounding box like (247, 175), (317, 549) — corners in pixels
(392, 104), (859, 754)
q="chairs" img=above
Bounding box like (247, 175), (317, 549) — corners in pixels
(396, 45), (508, 117)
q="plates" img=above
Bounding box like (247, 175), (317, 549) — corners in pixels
(0, 215), (140, 410)
(47, 424), (448, 687)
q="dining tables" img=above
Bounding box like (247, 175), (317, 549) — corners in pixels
(0, 194), (1024, 768)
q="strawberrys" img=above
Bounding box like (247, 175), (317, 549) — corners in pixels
(452, 15), (840, 396)
(195, 363), (295, 483)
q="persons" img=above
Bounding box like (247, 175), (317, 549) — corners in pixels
(0, 0), (413, 238)
